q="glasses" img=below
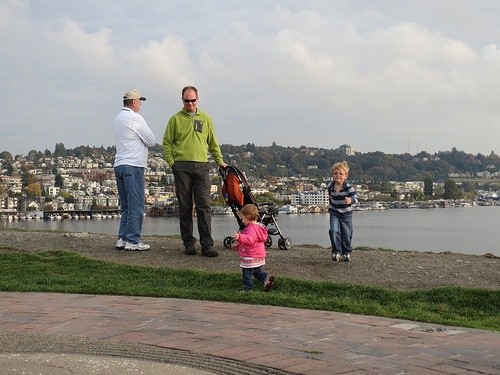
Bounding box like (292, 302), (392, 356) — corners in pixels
(183, 99), (196, 103)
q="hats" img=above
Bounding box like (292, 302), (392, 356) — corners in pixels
(123, 89), (147, 101)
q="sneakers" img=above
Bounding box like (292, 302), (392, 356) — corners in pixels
(115, 237), (126, 249)
(201, 245), (219, 257)
(124, 241), (150, 251)
(184, 239), (195, 255)
(263, 275), (275, 292)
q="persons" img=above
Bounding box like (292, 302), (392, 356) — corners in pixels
(113, 88), (157, 250)
(327, 162), (358, 262)
(231, 203), (275, 292)
(163, 86), (227, 257)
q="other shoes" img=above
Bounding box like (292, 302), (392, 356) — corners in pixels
(332, 253), (340, 260)
(343, 254), (351, 261)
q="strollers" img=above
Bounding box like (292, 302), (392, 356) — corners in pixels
(219, 164), (293, 251)
